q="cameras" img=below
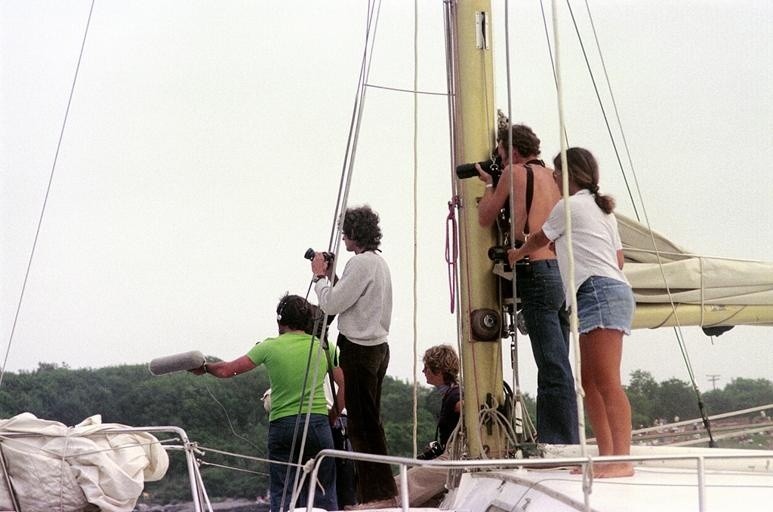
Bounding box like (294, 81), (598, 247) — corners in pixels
(304, 248), (334, 268)
(417, 442), (444, 460)
(457, 147), (503, 188)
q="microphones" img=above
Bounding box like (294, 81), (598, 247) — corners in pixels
(148, 351), (207, 375)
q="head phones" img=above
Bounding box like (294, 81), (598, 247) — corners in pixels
(277, 296), (298, 324)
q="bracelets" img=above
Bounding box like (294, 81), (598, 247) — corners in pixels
(485, 183), (494, 188)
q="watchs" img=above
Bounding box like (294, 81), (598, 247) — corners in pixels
(311, 274), (327, 283)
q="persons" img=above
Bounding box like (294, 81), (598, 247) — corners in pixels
(261, 304), (360, 509)
(186, 294), (344, 511)
(505, 147), (638, 479)
(311, 205), (398, 503)
(475, 124), (582, 445)
(392, 344), (464, 507)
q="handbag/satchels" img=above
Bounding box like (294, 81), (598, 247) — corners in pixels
(330, 426), (358, 507)
